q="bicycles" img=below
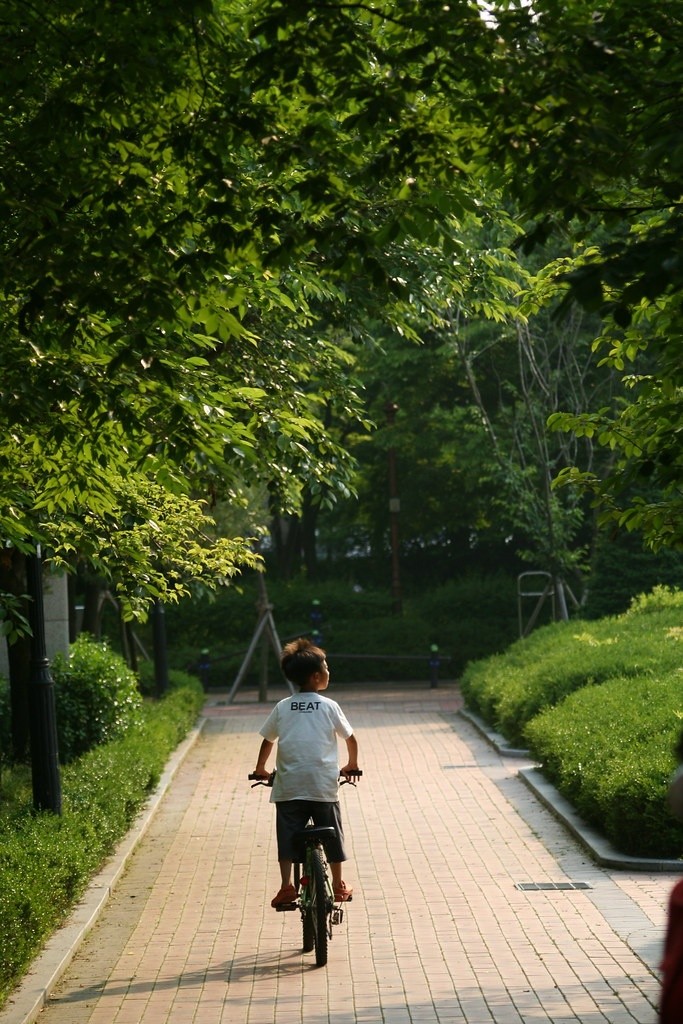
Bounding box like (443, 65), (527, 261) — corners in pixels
(248, 770), (363, 967)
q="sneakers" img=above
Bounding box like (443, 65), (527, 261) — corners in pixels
(331, 881), (354, 903)
(271, 885), (297, 908)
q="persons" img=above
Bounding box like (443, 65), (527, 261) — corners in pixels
(253, 639), (360, 908)
(657, 730), (683, 1024)
